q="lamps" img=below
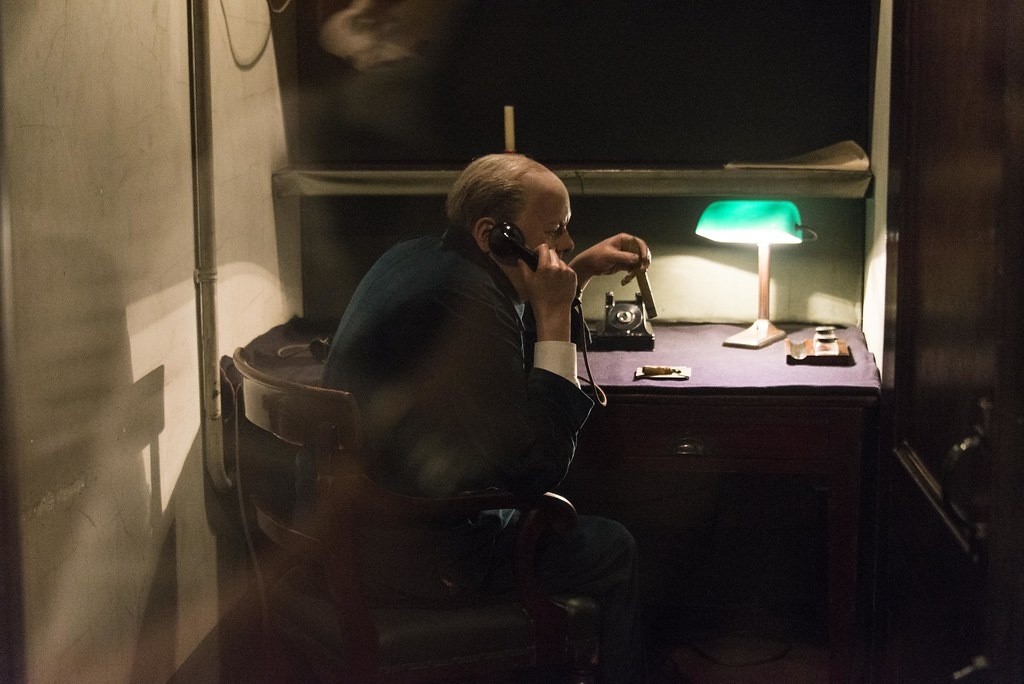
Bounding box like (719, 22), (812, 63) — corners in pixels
(695, 199), (819, 349)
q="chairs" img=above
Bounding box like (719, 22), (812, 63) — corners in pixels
(226, 351), (603, 684)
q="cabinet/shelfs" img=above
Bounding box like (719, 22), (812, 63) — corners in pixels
(269, 0), (902, 412)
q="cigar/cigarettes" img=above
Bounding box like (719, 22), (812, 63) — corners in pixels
(642, 365), (673, 375)
(636, 270), (657, 319)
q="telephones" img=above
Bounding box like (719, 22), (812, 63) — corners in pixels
(486, 220), (583, 311)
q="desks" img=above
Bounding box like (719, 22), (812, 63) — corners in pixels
(248, 314), (883, 684)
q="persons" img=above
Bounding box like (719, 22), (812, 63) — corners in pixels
(322, 153), (652, 683)
